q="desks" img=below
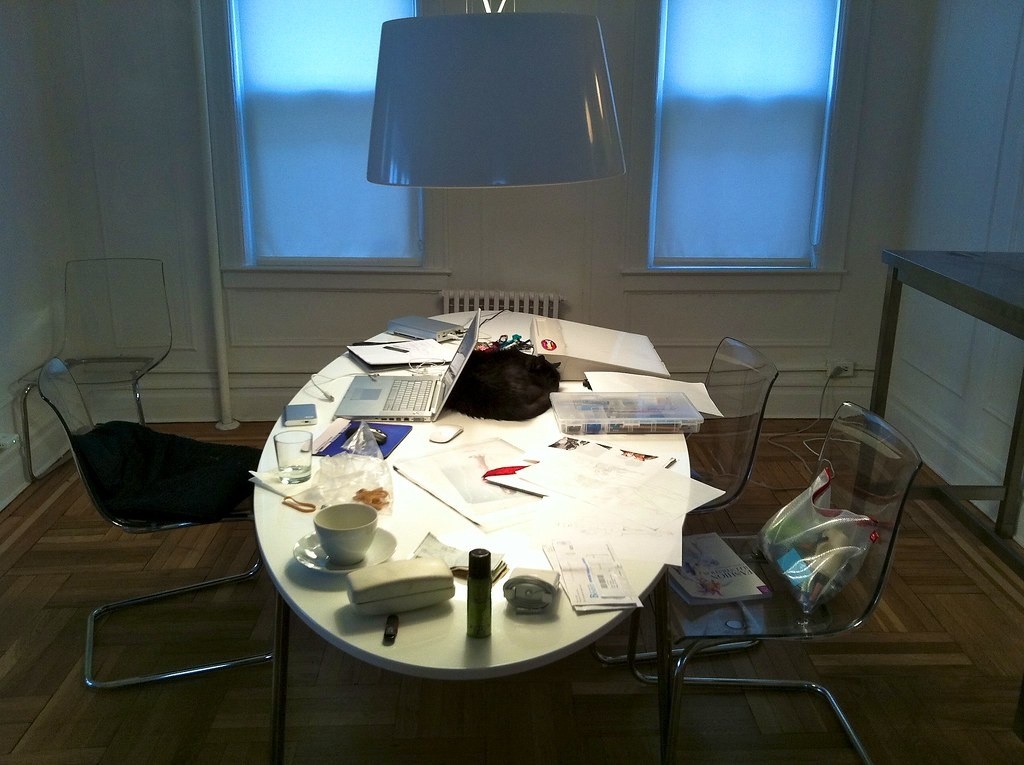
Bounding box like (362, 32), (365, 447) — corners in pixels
(850, 249), (1024, 581)
(253, 310), (690, 765)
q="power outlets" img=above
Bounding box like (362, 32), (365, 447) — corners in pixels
(827, 361), (854, 378)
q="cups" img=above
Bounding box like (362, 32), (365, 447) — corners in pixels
(273, 431), (313, 484)
(312, 503), (379, 565)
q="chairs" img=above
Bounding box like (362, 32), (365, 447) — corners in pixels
(18, 258), (172, 482)
(590, 337), (779, 662)
(627, 401), (923, 765)
(37, 356), (275, 694)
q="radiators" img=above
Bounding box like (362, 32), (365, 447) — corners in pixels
(433, 287), (567, 319)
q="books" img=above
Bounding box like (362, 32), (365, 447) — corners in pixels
(664, 532), (773, 607)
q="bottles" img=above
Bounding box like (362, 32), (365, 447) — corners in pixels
(466, 549), (492, 640)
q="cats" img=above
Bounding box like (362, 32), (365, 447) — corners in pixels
(443, 346), (561, 421)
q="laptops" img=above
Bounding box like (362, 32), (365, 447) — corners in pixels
(334, 308), (482, 421)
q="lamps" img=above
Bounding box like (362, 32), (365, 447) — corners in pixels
(367, 0), (626, 188)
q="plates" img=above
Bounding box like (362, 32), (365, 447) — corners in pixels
(292, 528), (397, 574)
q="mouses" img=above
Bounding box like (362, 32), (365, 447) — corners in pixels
(346, 428), (387, 446)
(429, 426), (464, 442)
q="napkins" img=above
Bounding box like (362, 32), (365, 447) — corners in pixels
(248, 466), (322, 497)
